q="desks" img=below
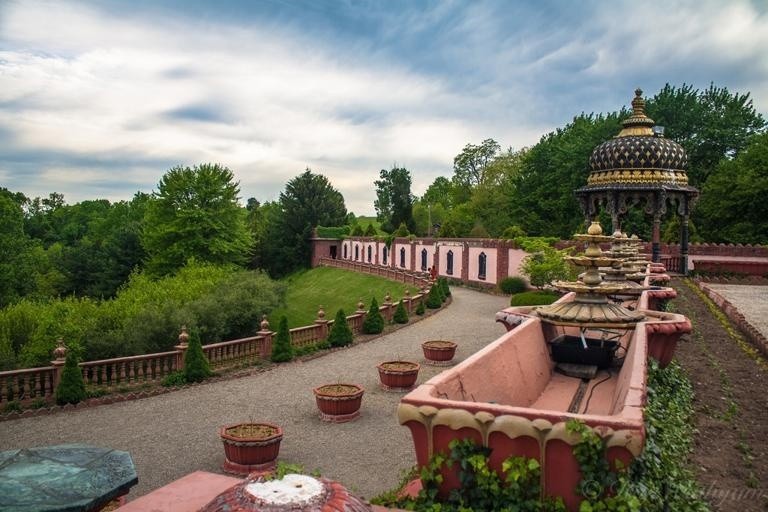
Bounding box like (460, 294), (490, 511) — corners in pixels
(0, 444), (140, 511)
